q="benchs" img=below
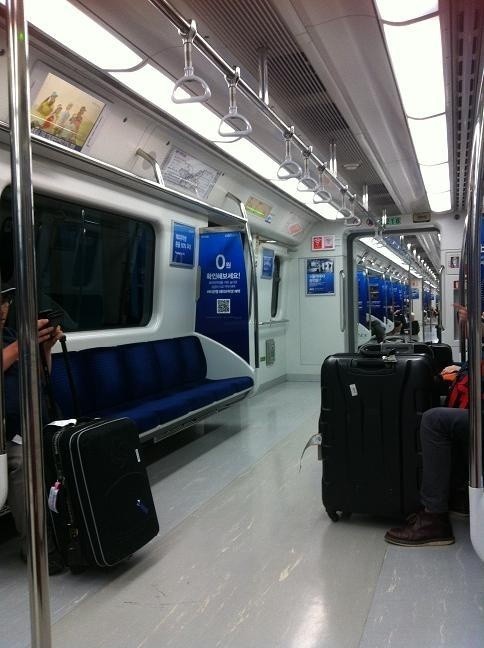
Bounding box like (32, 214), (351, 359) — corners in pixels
(0, 334), (254, 448)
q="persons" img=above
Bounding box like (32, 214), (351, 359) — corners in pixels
(408, 313), (420, 343)
(66, 106), (87, 141)
(0, 283), (74, 576)
(53, 103), (74, 135)
(450, 301), (468, 323)
(366, 320), (386, 344)
(381, 395), (472, 546)
(388, 307), (407, 336)
(41, 105), (62, 131)
(36, 92), (58, 116)
(403, 298), (410, 317)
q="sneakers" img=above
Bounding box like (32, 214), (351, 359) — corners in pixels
(21, 550), (66, 577)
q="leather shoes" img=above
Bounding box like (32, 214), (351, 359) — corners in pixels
(384, 512), (455, 547)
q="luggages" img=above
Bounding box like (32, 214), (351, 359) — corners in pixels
(36, 308), (160, 575)
(317, 341), (452, 522)
